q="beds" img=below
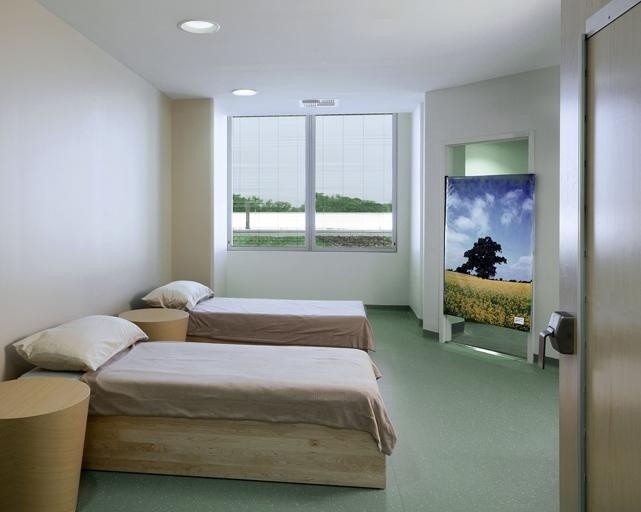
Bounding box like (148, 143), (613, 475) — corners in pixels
(134, 294), (371, 355)
(20, 339), (390, 493)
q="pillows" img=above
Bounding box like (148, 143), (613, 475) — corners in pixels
(138, 278), (215, 307)
(11, 313), (151, 375)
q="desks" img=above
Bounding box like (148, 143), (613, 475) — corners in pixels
(0, 376), (92, 511)
(118, 305), (191, 341)
